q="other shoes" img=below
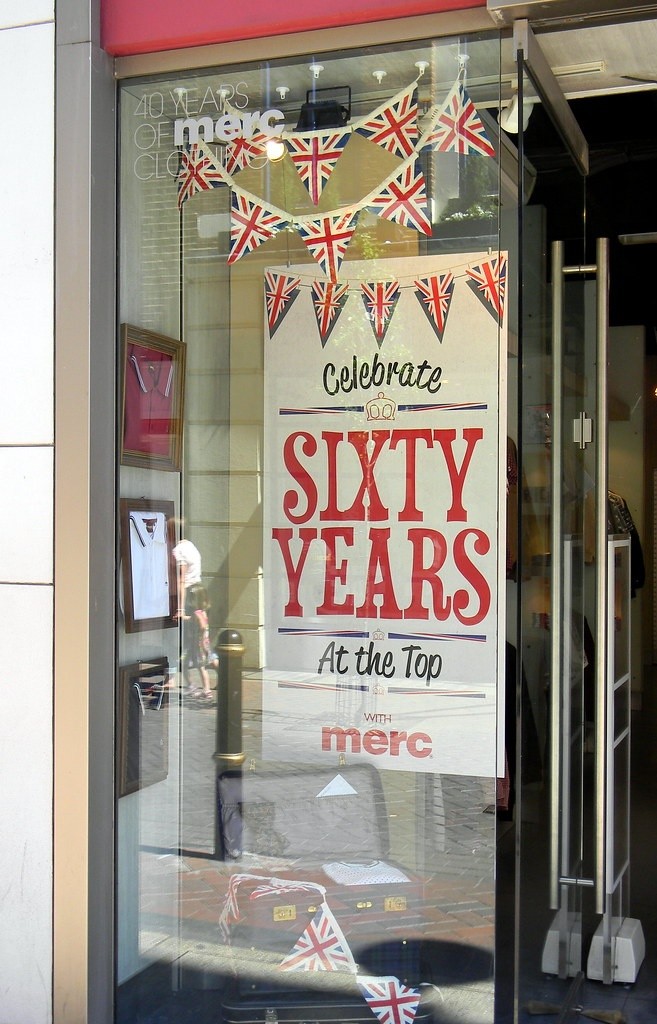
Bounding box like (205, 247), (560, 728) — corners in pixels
(183, 683), (197, 695)
(151, 683), (175, 691)
(197, 690), (213, 698)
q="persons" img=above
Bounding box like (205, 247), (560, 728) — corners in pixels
(151, 515), (219, 700)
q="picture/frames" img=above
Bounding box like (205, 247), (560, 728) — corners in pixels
(116, 324), (187, 472)
(121, 499), (179, 633)
(115, 656), (169, 799)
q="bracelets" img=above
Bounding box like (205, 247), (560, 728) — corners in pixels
(176, 609), (184, 610)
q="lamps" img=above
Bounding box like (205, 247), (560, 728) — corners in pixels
(497, 91), (535, 134)
(262, 135), (290, 163)
(291, 85), (355, 131)
